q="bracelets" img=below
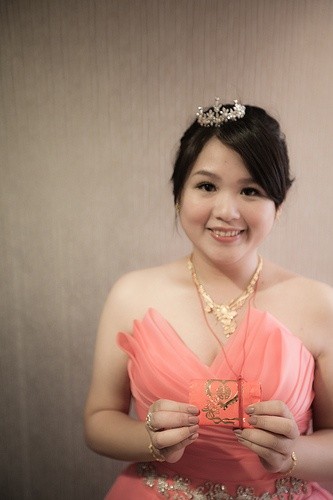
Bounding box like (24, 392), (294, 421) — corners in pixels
(148, 433), (166, 462)
(278, 449), (297, 476)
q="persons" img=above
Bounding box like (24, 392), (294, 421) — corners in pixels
(83, 103), (333, 500)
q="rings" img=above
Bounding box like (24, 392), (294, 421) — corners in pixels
(145, 412), (158, 431)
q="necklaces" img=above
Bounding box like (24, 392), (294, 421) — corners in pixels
(187, 251), (264, 335)
